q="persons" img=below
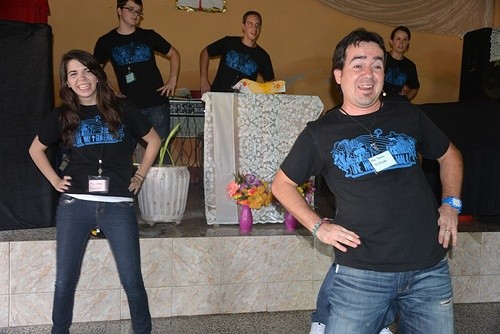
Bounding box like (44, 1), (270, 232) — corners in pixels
(94, 0), (180, 165)
(272, 28), (464, 334)
(200, 11), (274, 96)
(383, 26), (420, 100)
(28, 49), (161, 334)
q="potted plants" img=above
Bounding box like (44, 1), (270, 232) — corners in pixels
(138, 122), (189, 226)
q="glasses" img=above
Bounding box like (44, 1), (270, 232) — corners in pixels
(119, 7), (143, 17)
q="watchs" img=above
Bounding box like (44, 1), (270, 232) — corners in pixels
(442, 196), (462, 213)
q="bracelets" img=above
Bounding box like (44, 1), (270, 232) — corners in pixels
(134, 176), (141, 182)
(313, 218), (328, 236)
(136, 173), (144, 178)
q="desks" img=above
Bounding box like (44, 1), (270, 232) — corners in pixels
(200, 91), (324, 226)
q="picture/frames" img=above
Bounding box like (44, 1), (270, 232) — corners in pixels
(176, 0), (226, 13)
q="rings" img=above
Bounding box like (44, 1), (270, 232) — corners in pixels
(446, 230), (450, 232)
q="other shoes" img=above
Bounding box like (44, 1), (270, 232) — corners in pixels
(379, 328), (393, 334)
(309, 322), (326, 334)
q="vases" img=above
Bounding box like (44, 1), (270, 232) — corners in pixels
(285, 211), (298, 231)
(240, 205), (252, 232)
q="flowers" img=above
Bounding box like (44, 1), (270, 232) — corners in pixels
(226, 171), (275, 209)
(273, 180), (318, 215)
